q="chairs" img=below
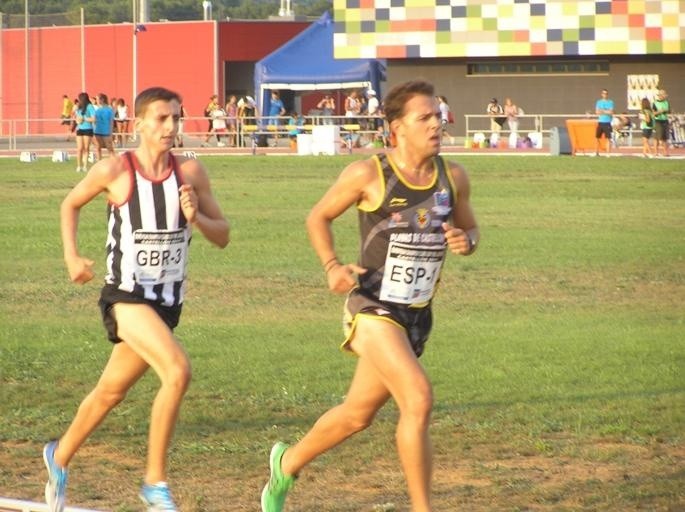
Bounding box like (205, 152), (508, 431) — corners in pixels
(465, 128), (544, 152)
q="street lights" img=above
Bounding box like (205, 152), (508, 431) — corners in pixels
(132, 24), (149, 145)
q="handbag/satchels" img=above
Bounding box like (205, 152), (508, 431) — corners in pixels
(447, 111), (453, 124)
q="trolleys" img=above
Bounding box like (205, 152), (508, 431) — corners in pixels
(670, 118), (685, 149)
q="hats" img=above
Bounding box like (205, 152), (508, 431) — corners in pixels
(655, 89), (666, 96)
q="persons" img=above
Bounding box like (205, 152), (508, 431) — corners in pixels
(434, 95), (452, 146)
(592, 87), (675, 159)
(61, 95), (130, 150)
(504, 97), (523, 148)
(486, 97), (504, 144)
(259, 82), (480, 510)
(173, 90), (391, 150)
(42, 87), (229, 512)
(92, 93), (118, 164)
(70, 93), (96, 175)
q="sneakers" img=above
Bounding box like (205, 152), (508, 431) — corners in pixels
(260, 441), (298, 512)
(136, 481), (176, 512)
(41, 441), (68, 511)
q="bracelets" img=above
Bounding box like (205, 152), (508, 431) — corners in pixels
(467, 236), (476, 251)
(326, 263), (340, 276)
(323, 257), (335, 268)
